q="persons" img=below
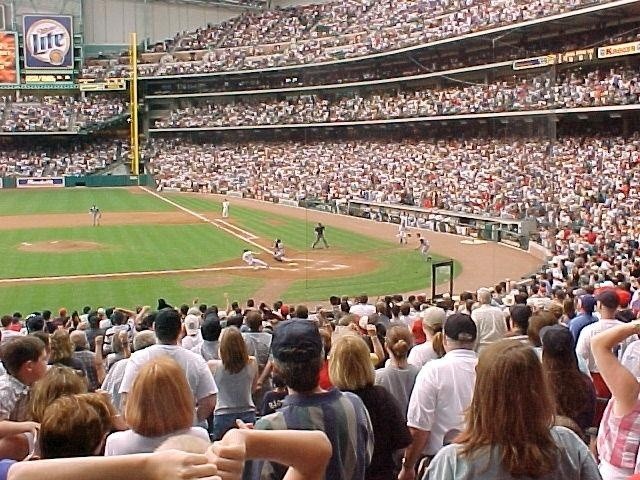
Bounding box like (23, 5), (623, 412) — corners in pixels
(1, 0), (640, 480)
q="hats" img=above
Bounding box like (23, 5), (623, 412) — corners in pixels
(443, 312), (477, 343)
(154, 307), (180, 324)
(577, 293), (597, 310)
(509, 304), (531, 323)
(593, 290), (621, 308)
(200, 312), (221, 340)
(538, 323), (575, 352)
(270, 317), (323, 363)
